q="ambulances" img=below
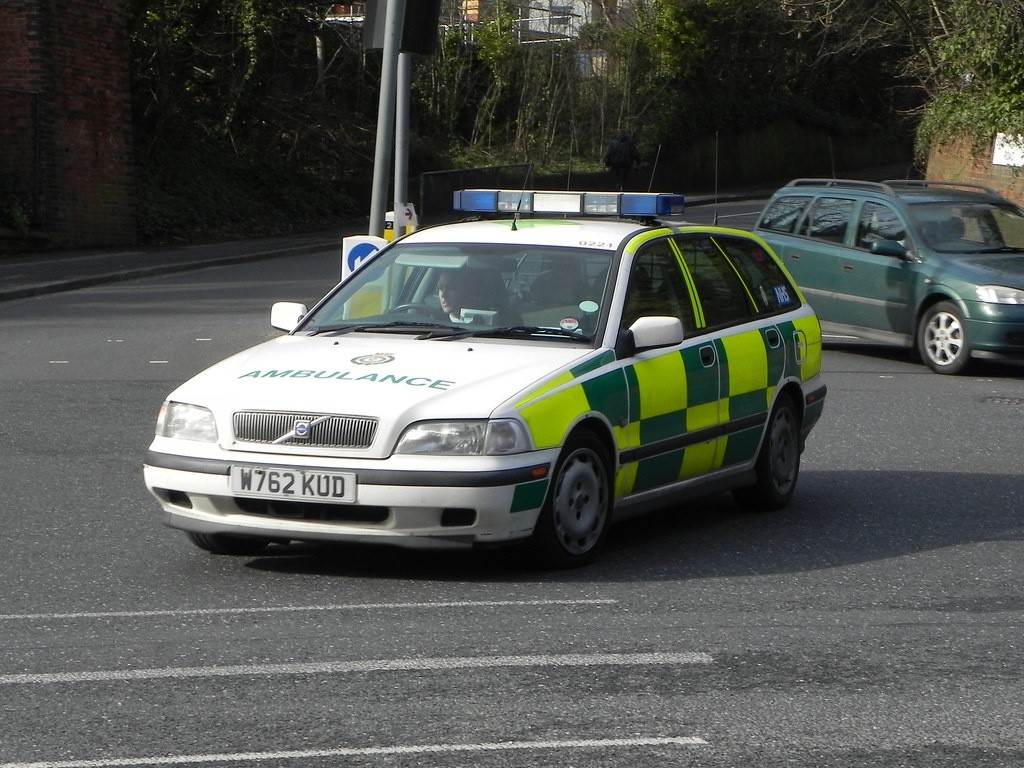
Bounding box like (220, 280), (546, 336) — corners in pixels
(143, 188), (827, 572)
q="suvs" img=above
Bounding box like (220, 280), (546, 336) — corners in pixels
(751, 179), (1024, 377)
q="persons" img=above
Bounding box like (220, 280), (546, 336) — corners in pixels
(431, 270), (482, 323)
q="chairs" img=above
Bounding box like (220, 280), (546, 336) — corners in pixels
(530, 272), (566, 303)
(591, 264), (651, 296)
(687, 272), (718, 302)
(937, 216), (966, 241)
